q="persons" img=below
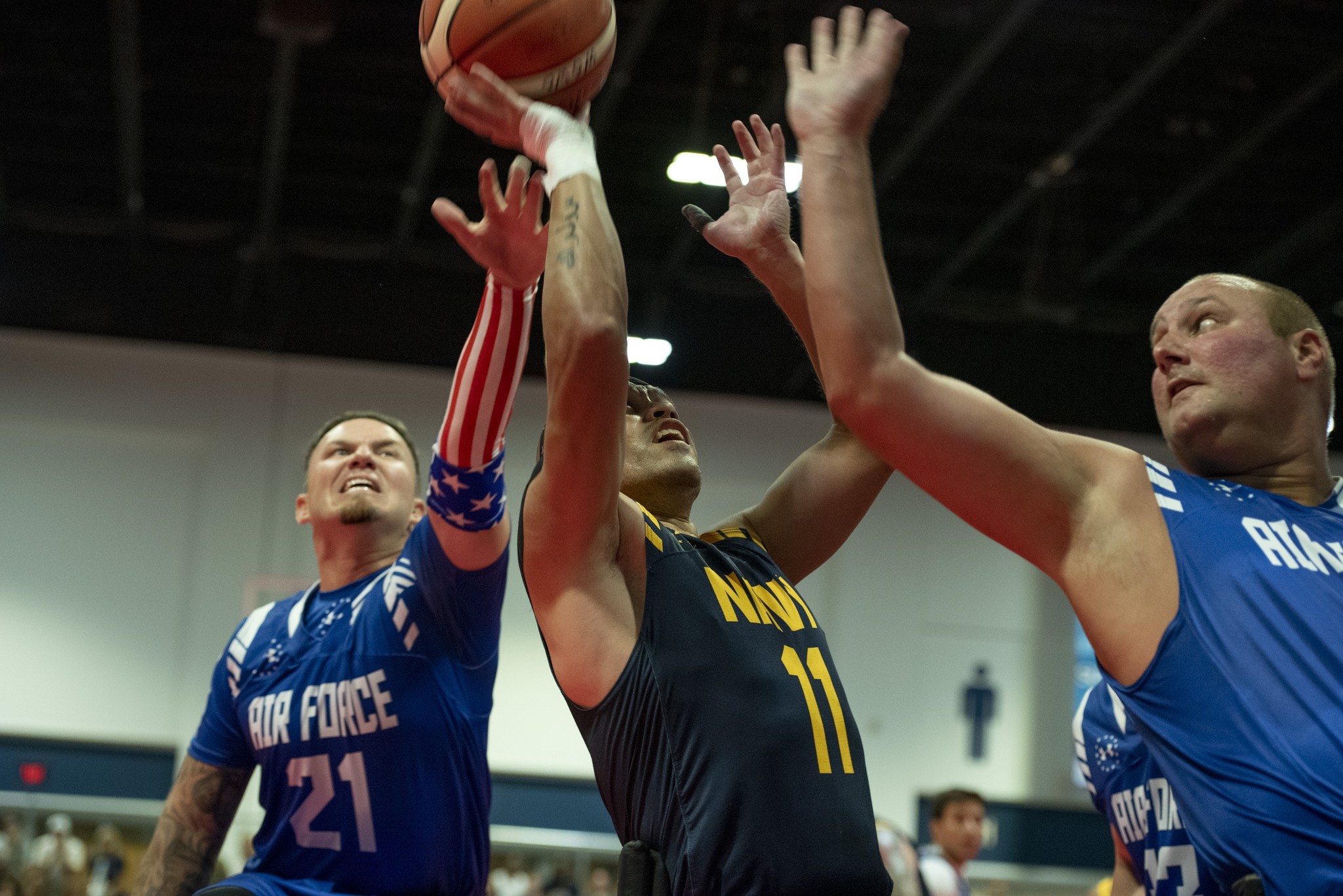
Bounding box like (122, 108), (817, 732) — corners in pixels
(137, 157), (551, 895)
(438, 60), (901, 896)
(784, 8), (1343, 896)
(1, 785), (1252, 895)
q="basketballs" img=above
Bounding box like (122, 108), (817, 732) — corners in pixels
(420, 0), (619, 116)
